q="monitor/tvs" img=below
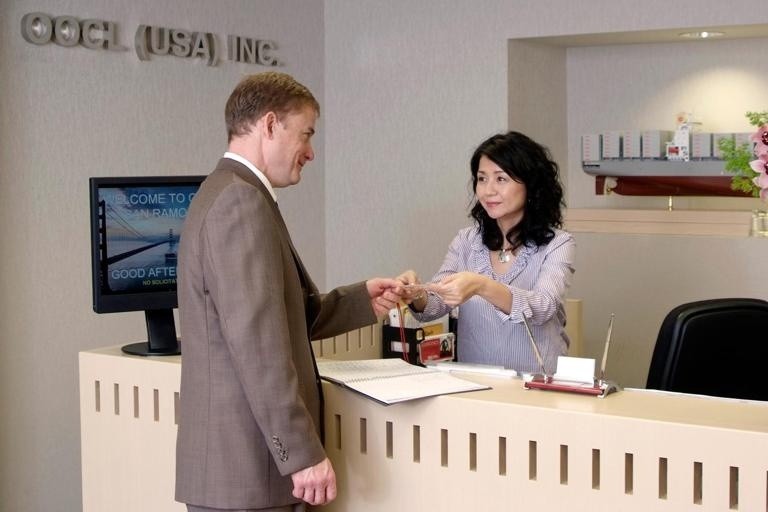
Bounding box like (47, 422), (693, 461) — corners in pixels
(88, 175), (210, 356)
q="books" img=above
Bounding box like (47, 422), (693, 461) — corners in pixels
(314, 357), (493, 407)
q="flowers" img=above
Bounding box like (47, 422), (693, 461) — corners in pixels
(717, 111), (768, 203)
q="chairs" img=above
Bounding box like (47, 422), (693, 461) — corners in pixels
(644, 299), (767, 402)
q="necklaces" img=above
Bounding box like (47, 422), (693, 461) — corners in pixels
(496, 236), (516, 263)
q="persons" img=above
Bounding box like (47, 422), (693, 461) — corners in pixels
(173, 71), (405, 511)
(395, 130), (576, 375)
